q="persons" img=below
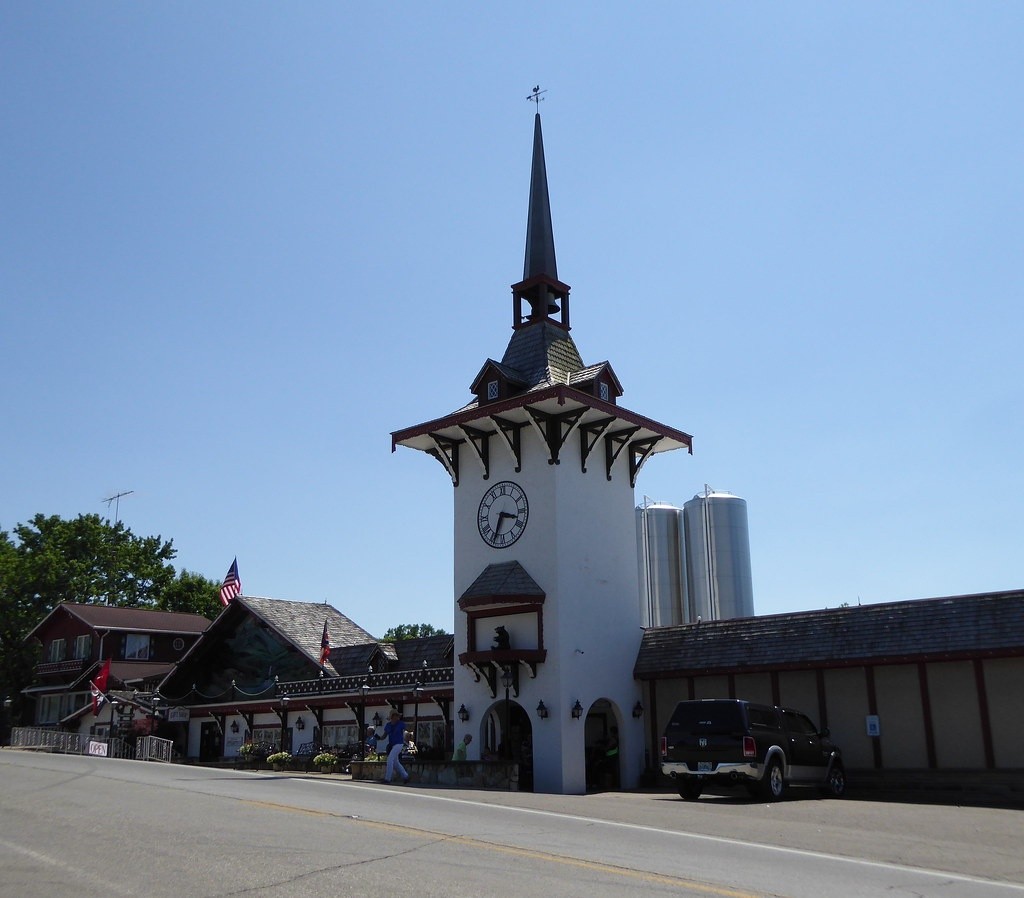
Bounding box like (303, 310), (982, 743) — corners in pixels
(366, 709), (412, 784)
(451, 734), (473, 760)
(480, 744), (508, 761)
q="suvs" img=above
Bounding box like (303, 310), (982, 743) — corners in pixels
(660, 698), (847, 805)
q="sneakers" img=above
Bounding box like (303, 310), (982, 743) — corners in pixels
(382, 779), (391, 784)
(400, 780), (411, 783)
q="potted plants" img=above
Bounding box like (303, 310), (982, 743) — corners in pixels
(313, 753), (338, 773)
(238, 740), (258, 760)
(267, 752), (292, 771)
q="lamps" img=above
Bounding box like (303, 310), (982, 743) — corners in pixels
(230, 720), (239, 734)
(457, 704), (469, 722)
(296, 716), (304, 731)
(536, 700), (548, 720)
(572, 699), (583, 720)
(633, 701), (644, 719)
(372, 712), (382, 726)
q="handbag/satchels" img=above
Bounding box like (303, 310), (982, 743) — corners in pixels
(605, 744), (619, 759)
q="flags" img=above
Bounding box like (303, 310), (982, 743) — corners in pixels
(89, 658), (111, 717)
(319, 618), (330, 670)
(218, 555), (242, 610)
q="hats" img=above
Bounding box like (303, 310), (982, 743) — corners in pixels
(464, 733), (472, 740)
(386, 709), (402, 722)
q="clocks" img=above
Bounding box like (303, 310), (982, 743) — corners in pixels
(477, 481), (528, 548)
